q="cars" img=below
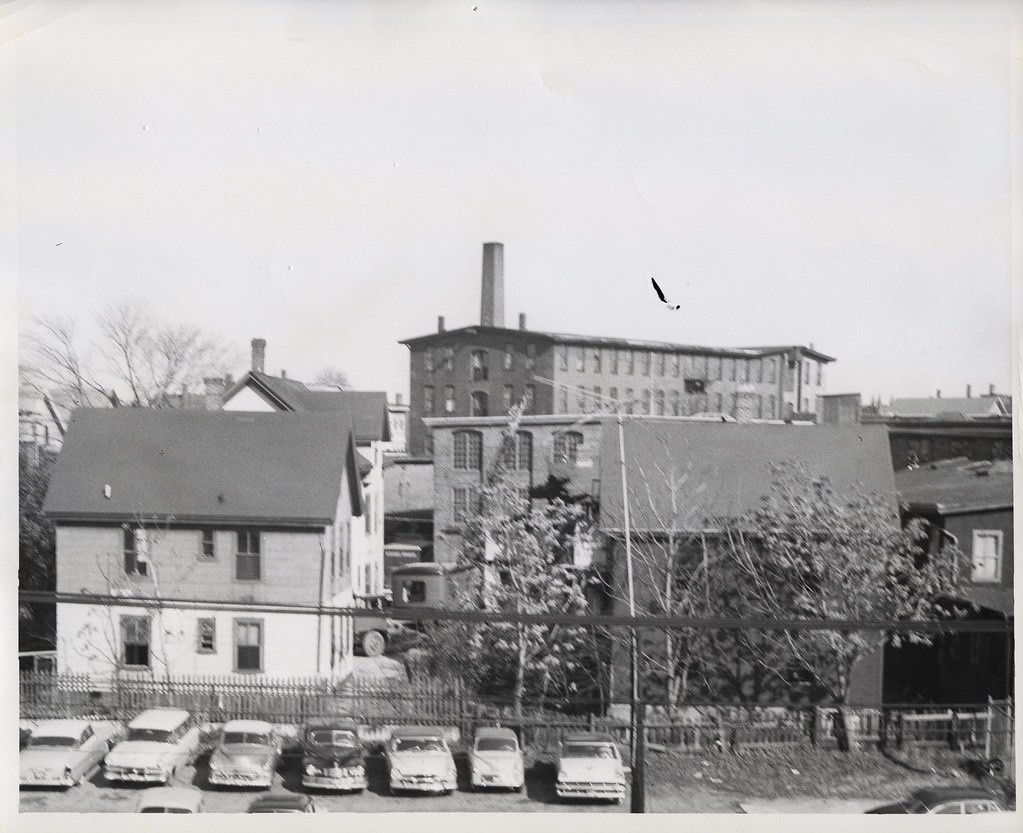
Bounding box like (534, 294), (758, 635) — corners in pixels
(467, 727), (524, 793)
(352, 616), (426, 656)
(19, 719), (104, 786)
(246, 794), (317, 813)
(136, 787), (203, 813)
(103, 708), (199, 782)
(207, 719), (282, 786)
(301, 720), (368, 789)
(557, 731), (631, 805)
(381, 727), (458, 795)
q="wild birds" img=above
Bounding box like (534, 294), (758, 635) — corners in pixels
(649, 276), (681, 310)
